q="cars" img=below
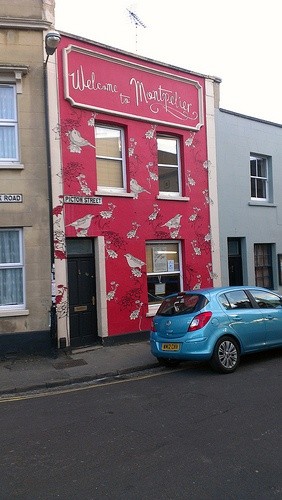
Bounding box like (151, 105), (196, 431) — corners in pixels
(147, 285), (282, 373)
